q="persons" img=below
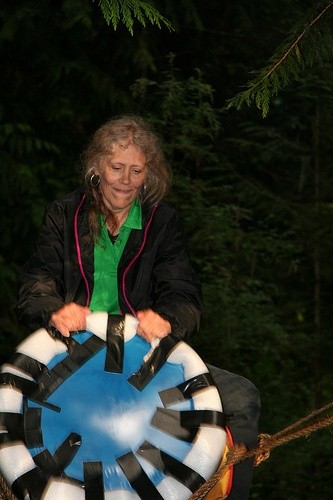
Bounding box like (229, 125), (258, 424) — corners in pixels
(12, 116), (264, 449)
